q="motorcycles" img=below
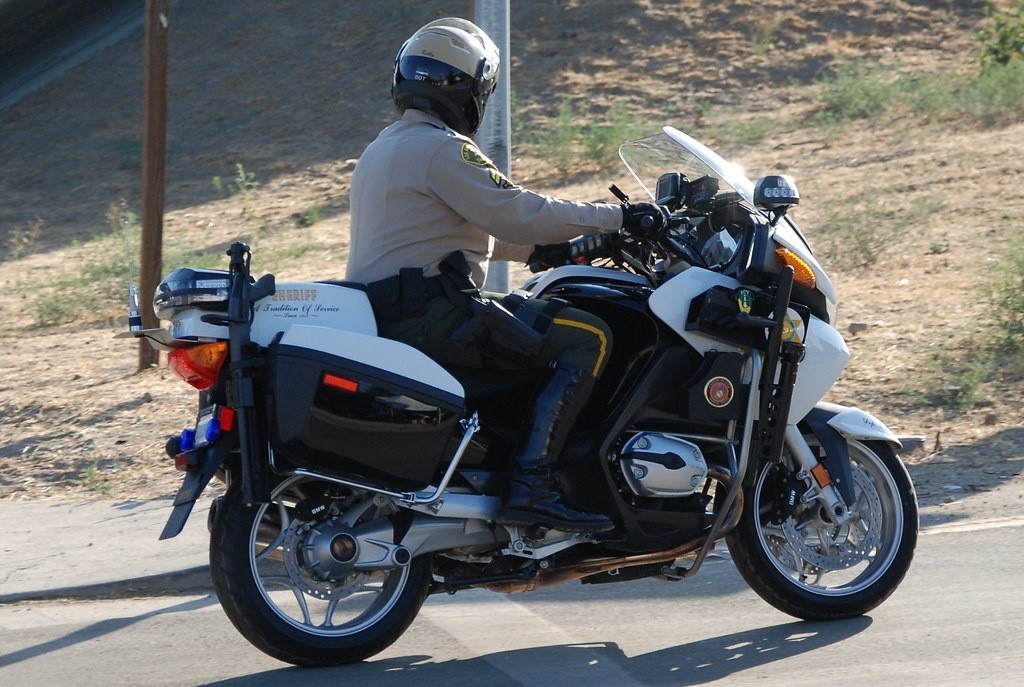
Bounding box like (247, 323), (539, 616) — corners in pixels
(126, 125), (928, 668)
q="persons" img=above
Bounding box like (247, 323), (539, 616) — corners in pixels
(346, 17), (670, 535)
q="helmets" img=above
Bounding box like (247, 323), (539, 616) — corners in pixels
(392, 17), (501, 137)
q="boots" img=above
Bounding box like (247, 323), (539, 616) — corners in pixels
(499, 361), (615, 533)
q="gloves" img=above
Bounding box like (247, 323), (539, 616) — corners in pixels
(620, 201), (667, 241)
(525, 242), (573, 269)
